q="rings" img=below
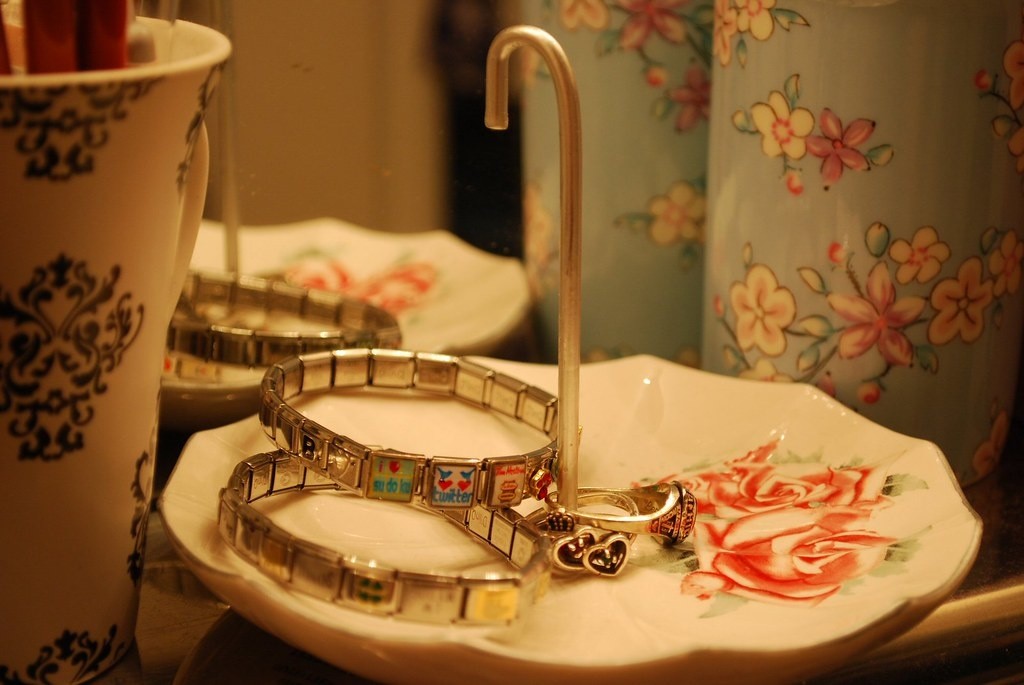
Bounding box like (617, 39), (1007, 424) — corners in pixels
(523, 492), (638, 577)
(544, 480), (696, 546)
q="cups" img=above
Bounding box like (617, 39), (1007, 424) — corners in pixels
(0, 13), (234, 685)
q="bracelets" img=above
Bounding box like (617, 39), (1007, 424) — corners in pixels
(168, 276), (402, 368)
(258, 347), (561, 510)
(218, 447), (551, 627)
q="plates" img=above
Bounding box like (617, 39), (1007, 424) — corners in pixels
(155, 353), (986, 685)
(157, 216), (530, 429)
